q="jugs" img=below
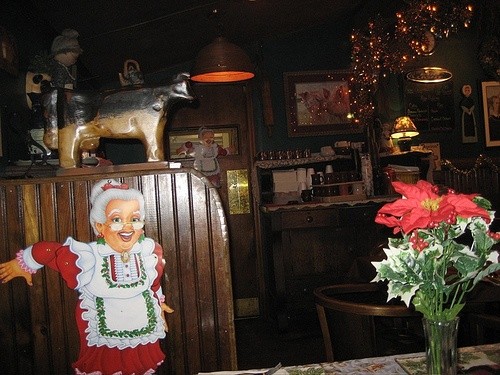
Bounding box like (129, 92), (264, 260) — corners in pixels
(311, 141), (363, 162)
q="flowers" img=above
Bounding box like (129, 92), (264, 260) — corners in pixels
(374, 179), (500, 375)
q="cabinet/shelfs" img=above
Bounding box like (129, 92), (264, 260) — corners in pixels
(262, 195), (406, 340)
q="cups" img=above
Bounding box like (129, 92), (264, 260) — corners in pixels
(257, 149), (310, 160)
(297, 165), (366, 203)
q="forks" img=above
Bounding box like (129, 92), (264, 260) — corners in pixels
(236, 363), (282, 375)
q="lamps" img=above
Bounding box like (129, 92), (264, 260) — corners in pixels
(190, 5), (256, 85)
(404, 40), (453, 83)
(391, 116), (420, 152)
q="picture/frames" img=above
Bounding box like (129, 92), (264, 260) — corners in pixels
(481, 82), (500, 147)
(401, 77), (458, 133)
(283, 71), (364, 138)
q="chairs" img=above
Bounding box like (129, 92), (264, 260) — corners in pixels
(313, 284), (500, 363)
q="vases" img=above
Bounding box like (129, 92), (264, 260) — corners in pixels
(422, 315), (460, 375)
